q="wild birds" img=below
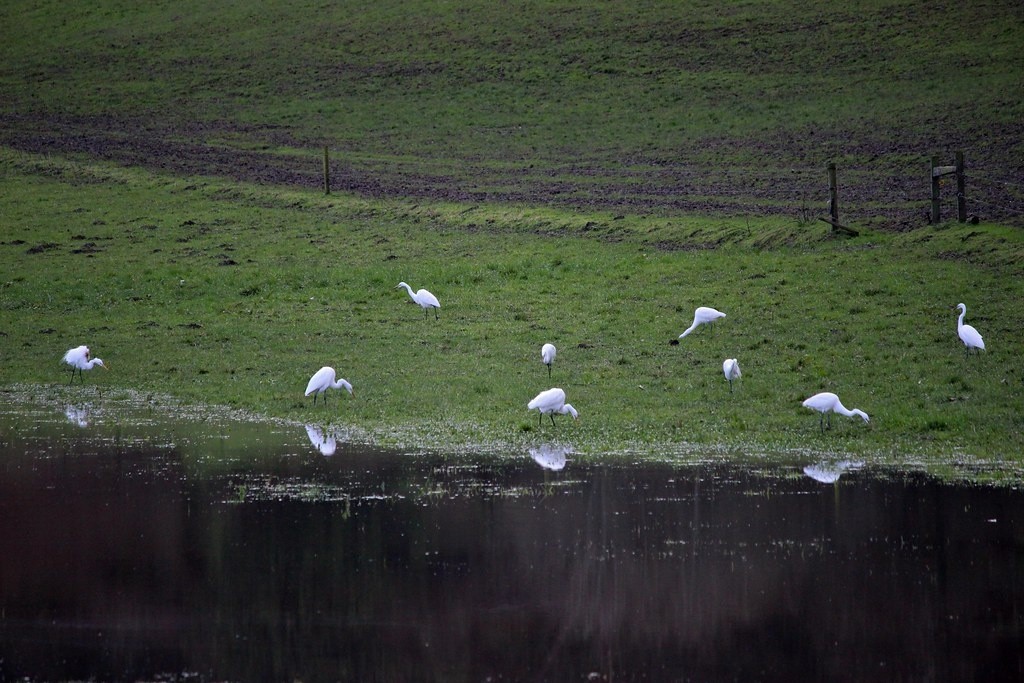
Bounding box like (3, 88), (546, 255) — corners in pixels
(541, 343), (557, 379)
(528, 441), (574, 471)
(802, 459), (866, 483)
(526, 387), (579, 426)
(953, 302), (986, 359)
(63, 400), (97, 428)
(723, 358), (742, 393)
(393, 281), (442, 321)
(59, 345), (109, 386)
(802, 392), (870, 435)
(668, 306), (727, 346)
(304, 365), (357, 408)
(304, 421), (336, 457)
